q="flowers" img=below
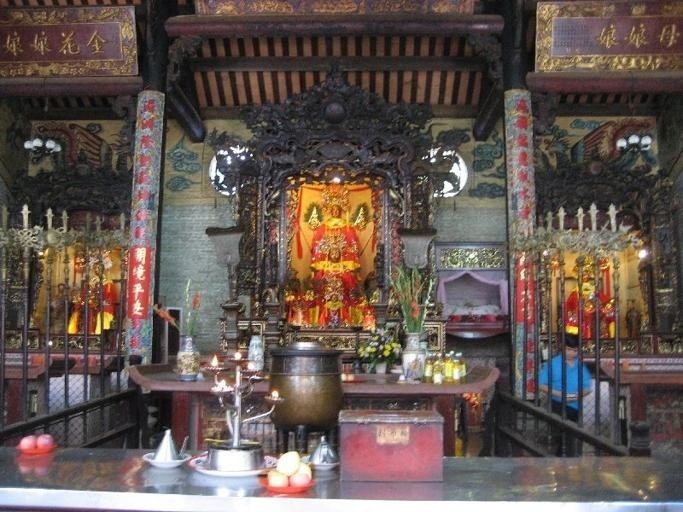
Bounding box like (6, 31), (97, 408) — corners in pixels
(149, 277), (202, 336)
(356, 334), (403, 362)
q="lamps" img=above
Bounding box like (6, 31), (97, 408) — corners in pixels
(203, 351), (284, 471)
(24, 97), (62, 163)
(615, 103), (652, 155)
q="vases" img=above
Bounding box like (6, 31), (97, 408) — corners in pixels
(176, 336), (199, 381)
(363, 361), (396, 374)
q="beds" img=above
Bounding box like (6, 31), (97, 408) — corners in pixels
(441, 304), (510, 339)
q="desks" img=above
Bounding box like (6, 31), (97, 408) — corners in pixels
(4, 351), (129, 449)
(583, 355), (682, 448)
(130, 362), (501, 456)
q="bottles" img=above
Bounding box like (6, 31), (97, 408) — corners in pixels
(424, 351), (468, 386)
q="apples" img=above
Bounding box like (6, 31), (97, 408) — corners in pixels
(19, 434), (53, 448)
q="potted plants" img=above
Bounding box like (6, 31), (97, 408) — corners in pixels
(384, 263), (432, 380)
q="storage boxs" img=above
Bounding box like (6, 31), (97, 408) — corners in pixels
(339, 410), (445, 483)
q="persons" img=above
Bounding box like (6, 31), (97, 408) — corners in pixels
(67, 250), (116, 335)
(539, 334), (592, 457)
(289, 185), (376, 328)
(565, 256), (616, 339)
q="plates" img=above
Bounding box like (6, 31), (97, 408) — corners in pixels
(15, 442), (58, 456)
(298, 456), (340, 471)
(258, 476), (318, 494)
(189, 455), (282, 478)
(141, 453), (191, 469)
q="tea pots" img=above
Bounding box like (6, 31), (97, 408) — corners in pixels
(305, 434), (339, 465)
(150, 429), (189, 462)
